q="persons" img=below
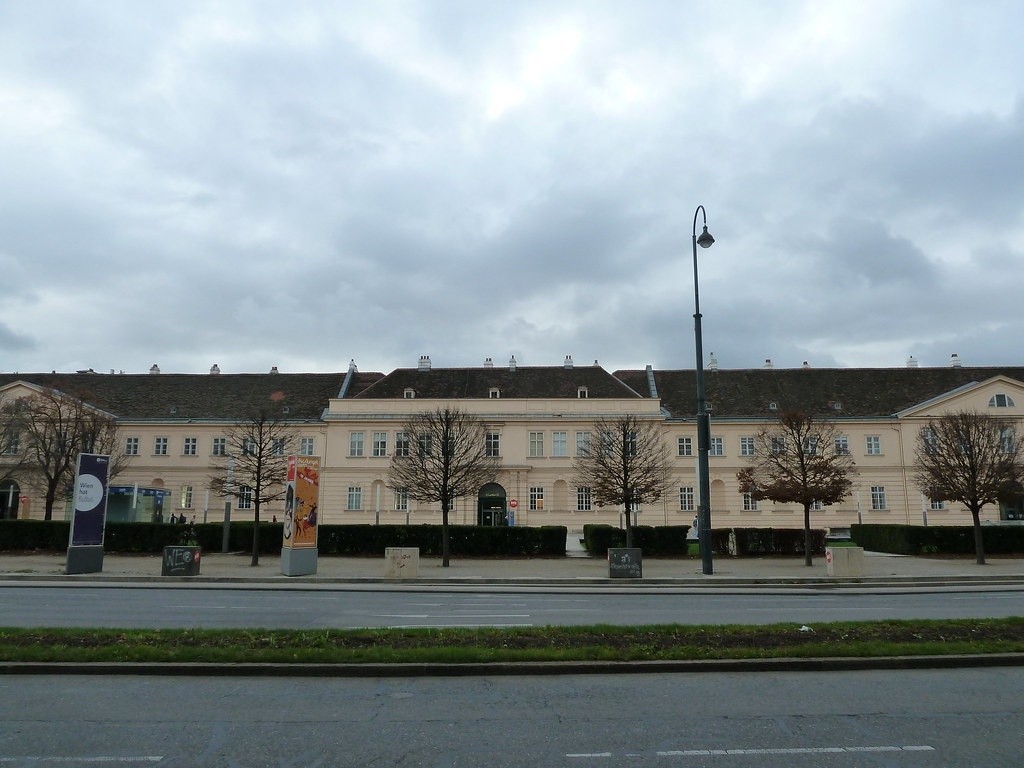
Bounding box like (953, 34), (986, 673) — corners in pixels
(179, 513), (184, 524)
(273, 515), (276, 522)
(191, 515), (196, 525)
(171, 514), (177, 523)
